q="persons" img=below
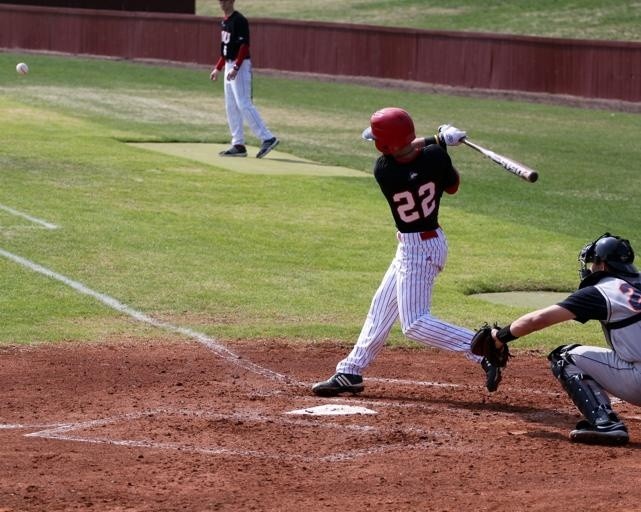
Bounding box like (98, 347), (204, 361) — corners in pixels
(207, 1), (281, 160)
(311, 106), (503, 398)
(471, 229), (641, 446)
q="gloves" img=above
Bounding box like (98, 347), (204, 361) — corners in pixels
(433, 125), (467, 146)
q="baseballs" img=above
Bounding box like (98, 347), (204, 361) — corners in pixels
(16, 62), (28, 76)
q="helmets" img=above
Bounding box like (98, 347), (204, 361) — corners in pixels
(577, 232), (635, 282)
(362, 107), (416, 155)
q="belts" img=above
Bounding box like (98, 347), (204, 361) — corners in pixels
(418, 230), (438, 242)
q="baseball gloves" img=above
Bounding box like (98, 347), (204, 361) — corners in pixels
(470, 321), (516, 371)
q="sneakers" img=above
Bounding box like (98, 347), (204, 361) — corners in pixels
(256, 137), (280, 159)
(481, 356), (502, 393)
(311, 373), (364, 395)
(219, 145), (247, 157)
(569, 419), (629, 446)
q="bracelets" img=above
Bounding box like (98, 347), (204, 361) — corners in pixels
(234, 65), (239, 70)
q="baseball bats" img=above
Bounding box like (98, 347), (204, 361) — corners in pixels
(438, 126), (538, 183)
